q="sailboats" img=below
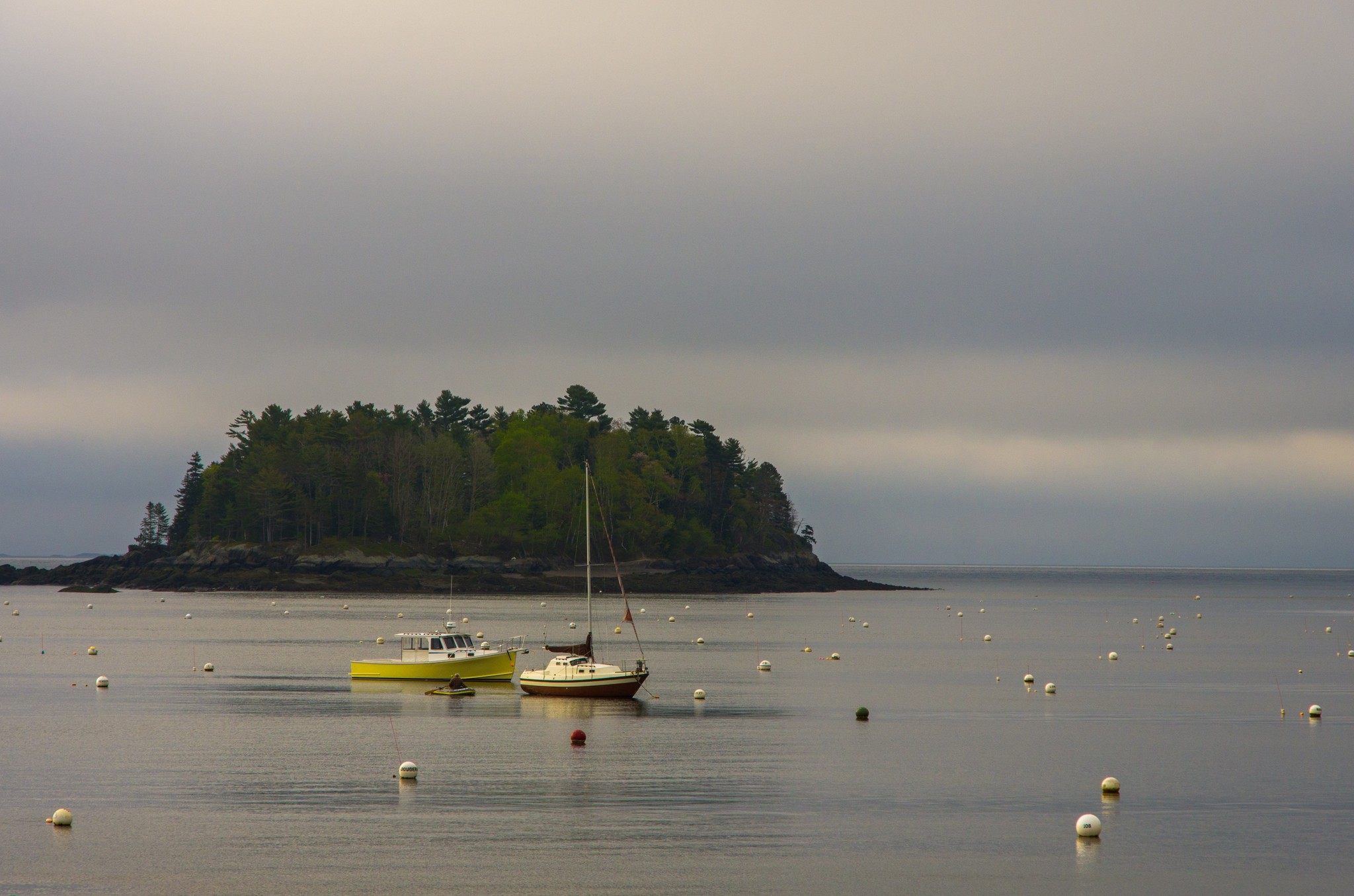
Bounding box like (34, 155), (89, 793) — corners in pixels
(519, 459), (650, 699)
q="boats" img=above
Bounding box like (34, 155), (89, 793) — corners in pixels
(348, 575), (529, 680)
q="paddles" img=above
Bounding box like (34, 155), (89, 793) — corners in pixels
(423, 684), (449, 695)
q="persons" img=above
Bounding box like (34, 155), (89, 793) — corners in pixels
(449, 673), (467, 689)
